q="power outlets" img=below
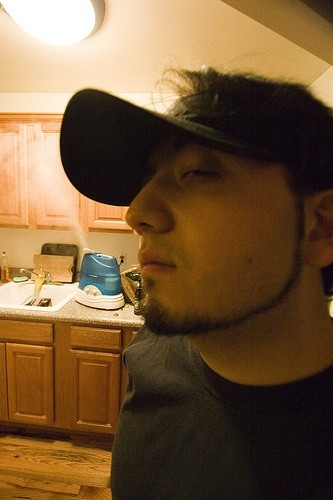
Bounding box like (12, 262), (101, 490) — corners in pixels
(117, 252), (128, 267)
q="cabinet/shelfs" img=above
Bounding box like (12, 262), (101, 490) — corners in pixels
(0, 317), (142, 447)
(0, 112), (139, 235)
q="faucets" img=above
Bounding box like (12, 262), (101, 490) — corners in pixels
(19, 269), (51, 284)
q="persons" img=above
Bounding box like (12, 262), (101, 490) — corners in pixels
(61, 67), (333, 500)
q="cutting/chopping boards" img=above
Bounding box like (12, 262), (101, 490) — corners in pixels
(31, 255), (74, 282)
(41, 243), (78, 283)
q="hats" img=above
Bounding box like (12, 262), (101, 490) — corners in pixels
(60, 89), (333, 206)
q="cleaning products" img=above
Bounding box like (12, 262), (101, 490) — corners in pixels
(0, 252), (8, 283)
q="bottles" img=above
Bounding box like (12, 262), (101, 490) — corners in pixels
(134, 279), (144, 315)
(0, 252), (9, 283)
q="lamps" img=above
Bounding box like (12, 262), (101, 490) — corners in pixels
(0, 0), (108, 49)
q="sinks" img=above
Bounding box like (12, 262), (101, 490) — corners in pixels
(0, 283), (78, 312)
